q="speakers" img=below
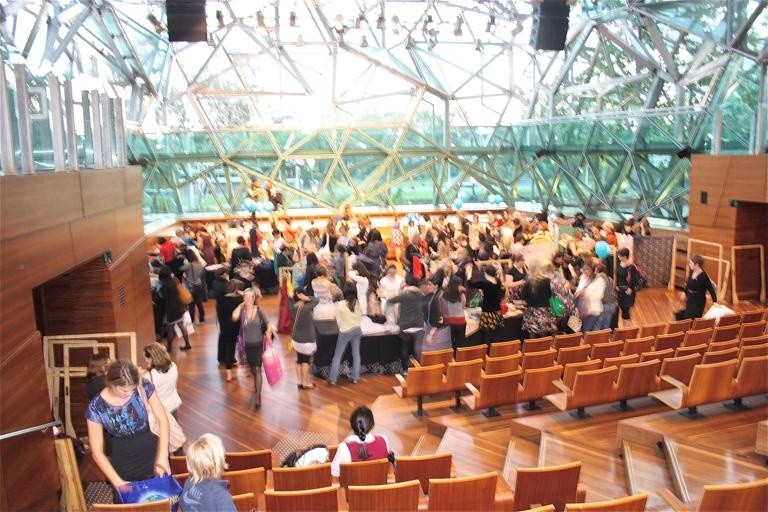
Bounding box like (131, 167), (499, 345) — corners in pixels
(166, 0), (207, 42)
(529, 0), (570, 51)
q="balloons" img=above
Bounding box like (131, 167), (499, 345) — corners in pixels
(488, 194), (496, 204)
(494, 194), (502, 205)
(244, 197), (275, 212)
(454, 198), (463, 210)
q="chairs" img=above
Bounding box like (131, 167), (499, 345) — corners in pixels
(392, 309), (767, 412)
(87, 448), (768, 510)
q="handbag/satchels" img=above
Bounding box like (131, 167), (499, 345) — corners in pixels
(262, 333), (283, 386)
(137, 378), (186, 454)
(114, 475), (183, 512)
(674, 298), (688, 321)
(627, 264), (645, 292)
(566, 314), (583, 333)
(173, 278), (194, 305)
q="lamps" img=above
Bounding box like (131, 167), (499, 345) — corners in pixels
(330, 0), (530, 60)
(143, 0), (301, 33)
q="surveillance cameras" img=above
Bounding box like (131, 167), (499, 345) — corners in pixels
(536, 149), (545, 158)
(676, 146), (691, 159)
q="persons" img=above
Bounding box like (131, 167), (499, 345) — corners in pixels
(245, 177), (262, 219)
(142, 343), (182, 426)
(265, 180), (283, 213)
(178, 433), (244, 512)
(88, 353), (118, 454)
(152, 208), (651, 386)
(311, 404), (392, 483)
(233, 288), (273, 409)
(84, 359), (170, 499)
(674, 254), (717, 320)
